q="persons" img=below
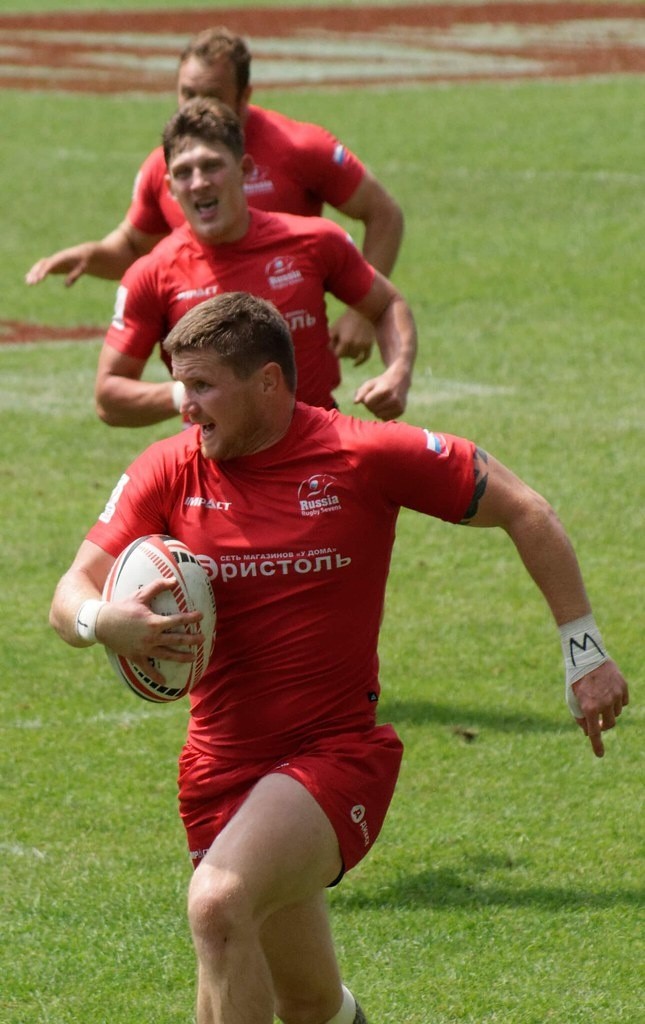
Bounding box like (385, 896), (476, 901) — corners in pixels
(91, 95), (431, 434)
(40, 298), (634, 1024)
(23, 18), (405, 366)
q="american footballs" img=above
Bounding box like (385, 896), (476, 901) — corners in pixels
(99, 533), (218, 705)
